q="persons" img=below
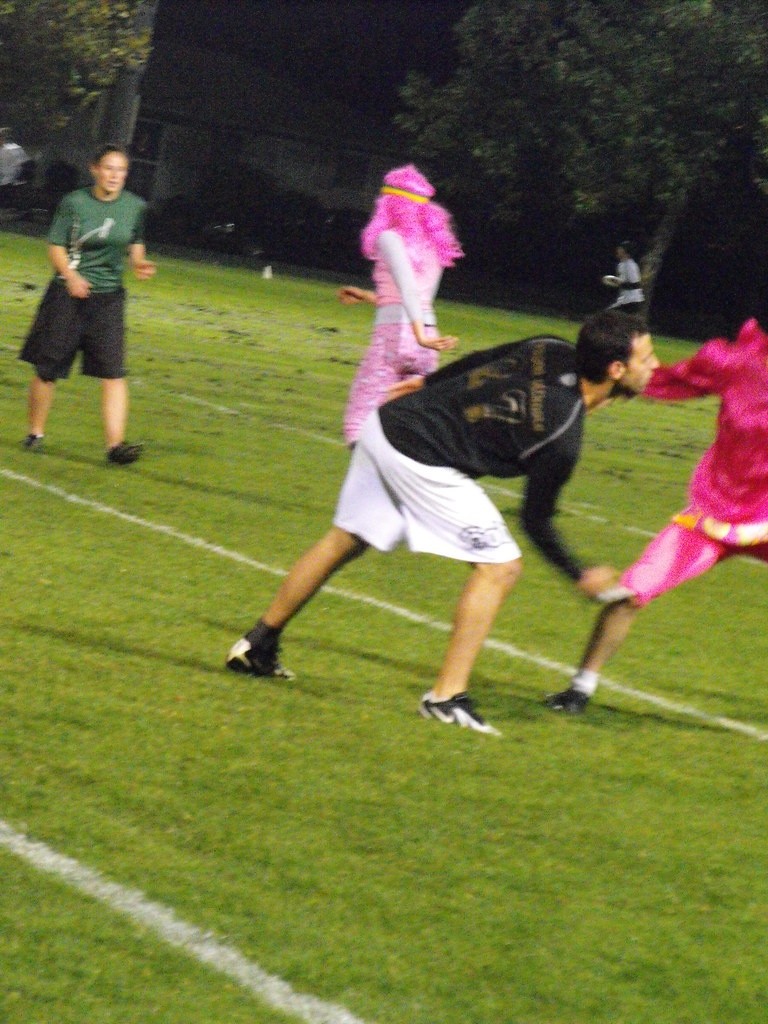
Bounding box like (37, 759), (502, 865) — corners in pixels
(15, 142), (156, 467)
(0, 127), (35, 222)
(538, 317), (768, 715)
(339, 163), (464, 452)
(602, 240), (645, 315)
(226, 307), (659, 736)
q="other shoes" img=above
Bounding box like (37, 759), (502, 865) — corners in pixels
(107, 442), (145, 464)
(19, 434), (43, 451)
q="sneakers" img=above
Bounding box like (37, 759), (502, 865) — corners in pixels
(226, 619), (298, 682)
(417, 691), (503, 736)
(536, 689), (590, 716)
